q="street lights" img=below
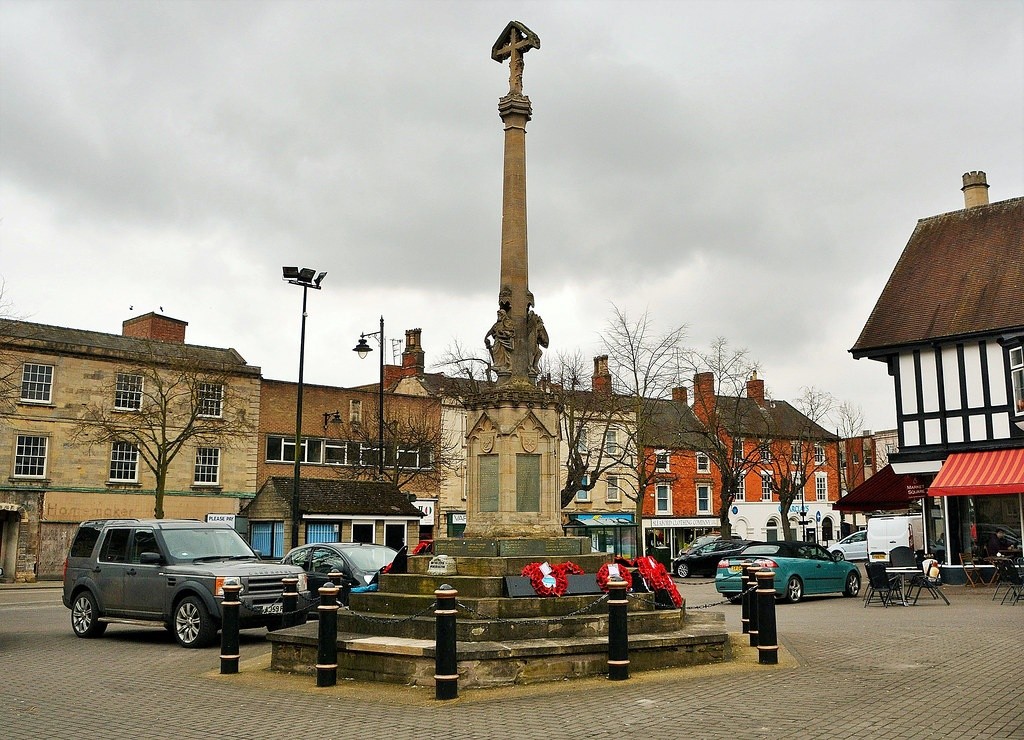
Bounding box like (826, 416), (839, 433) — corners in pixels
(351, 315), (383, 483)
(281, 265), (328, 550)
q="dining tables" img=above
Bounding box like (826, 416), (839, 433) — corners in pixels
(983, 555), (1014, 588)
(998, 549), (1021, 555)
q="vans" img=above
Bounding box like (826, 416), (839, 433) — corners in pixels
(867, 513), (924, 563)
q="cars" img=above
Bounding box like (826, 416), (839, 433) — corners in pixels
(279, 541), (400, 612)
(671, 540), (761, 577)
(678, 532), (742, 556)
(963, 521), (1022, 551)
(824, 530), (869, 562)
(715, 540), (863, 605)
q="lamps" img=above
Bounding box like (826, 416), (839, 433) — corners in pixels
(323, 410), (344, 430)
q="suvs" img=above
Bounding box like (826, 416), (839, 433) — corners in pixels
(61, 516), (310, 647)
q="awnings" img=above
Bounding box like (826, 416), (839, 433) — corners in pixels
(928, 448), (1024, 496)
(575, 514), (638, 526)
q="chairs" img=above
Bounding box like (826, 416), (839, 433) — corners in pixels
(959, 552), (986, 588)
(991, 559), (1024, 606)
(862, 545), (950, 607)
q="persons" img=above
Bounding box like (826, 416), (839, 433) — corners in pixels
(937, 533), (945, 546)
(985, 528), (1012, 556)
